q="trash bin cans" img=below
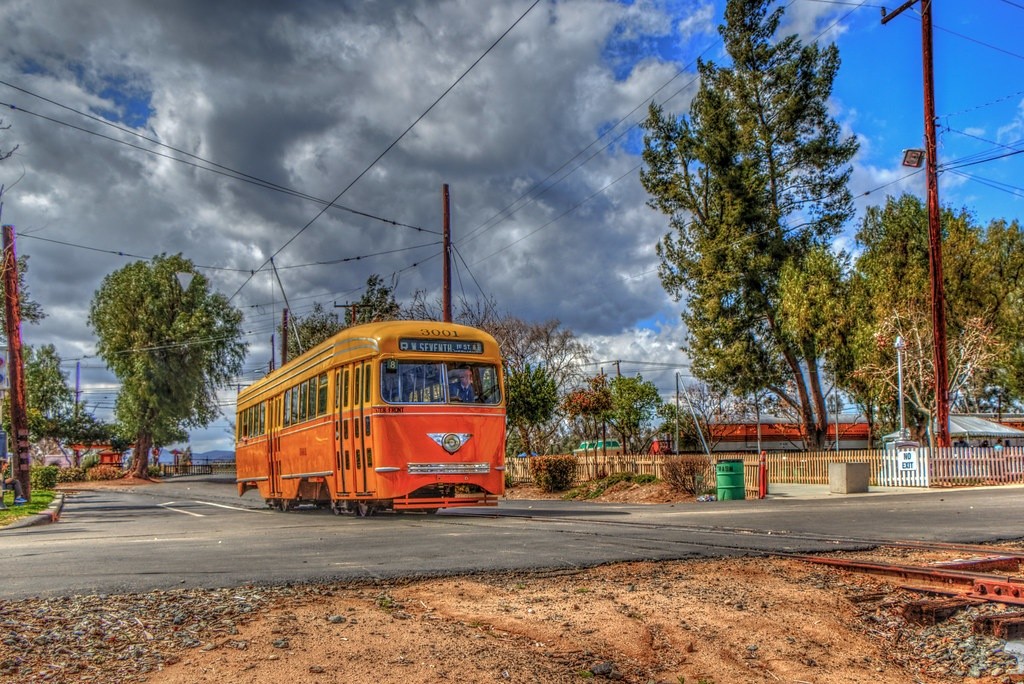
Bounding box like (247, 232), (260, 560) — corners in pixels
(716, 458), (745, 501)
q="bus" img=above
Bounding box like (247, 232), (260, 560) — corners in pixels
(235, 318), (507, 517)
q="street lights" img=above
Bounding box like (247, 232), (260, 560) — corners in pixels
(895, 335), (908, 442)
